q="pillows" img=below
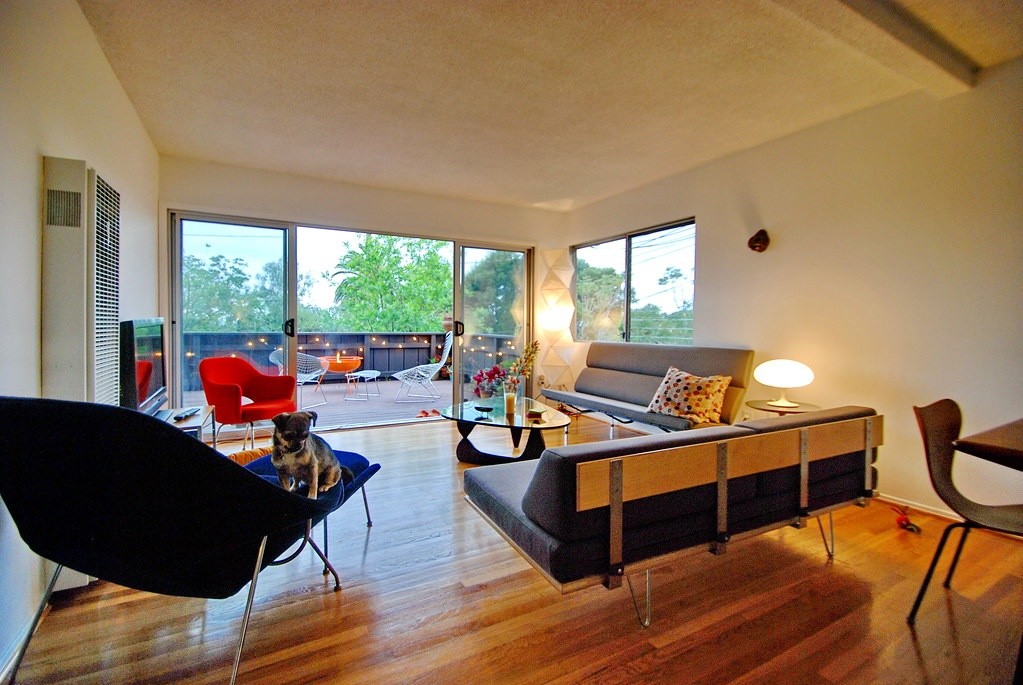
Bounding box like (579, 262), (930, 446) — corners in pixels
(646, 365), (732, 423)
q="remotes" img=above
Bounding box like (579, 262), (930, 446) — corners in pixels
(173, 408), (200, 421)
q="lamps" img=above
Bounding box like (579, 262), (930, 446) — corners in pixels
(753, 357), (815, 408)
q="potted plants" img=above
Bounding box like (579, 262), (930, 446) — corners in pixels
(447, 363), (465, 384)
(479, 384), (492, 399)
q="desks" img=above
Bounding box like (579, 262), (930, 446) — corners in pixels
(315, 356), (364, 396)
(344, 370), (381, 400)
(746, 400), (823, 416)
(166, 405), (217, 449)
(951, 418), (1023, 471)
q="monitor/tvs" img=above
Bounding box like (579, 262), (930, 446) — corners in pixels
(120, 317), (174, 423)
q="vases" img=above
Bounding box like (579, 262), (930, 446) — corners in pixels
(505, 392), (515, 415)
(427, 368), (449, 380)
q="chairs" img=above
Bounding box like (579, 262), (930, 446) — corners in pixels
(0, 395), (422, 685)
(906, 397), (1023, 625)
(198, 357), (297, 449)
(269, 349), (330, 409)
(391, 331), (453, 404)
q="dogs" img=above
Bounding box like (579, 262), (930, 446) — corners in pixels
(272, 410), (356, 500)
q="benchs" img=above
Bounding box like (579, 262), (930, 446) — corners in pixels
(462, 405), (884, 627)
(541, 341), (755, 440)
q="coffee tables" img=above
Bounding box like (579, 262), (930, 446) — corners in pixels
(440, 397), (572, 466)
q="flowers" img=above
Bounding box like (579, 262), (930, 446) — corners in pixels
(428, 355), (452, 367)
(472, 340), (541, 395)
